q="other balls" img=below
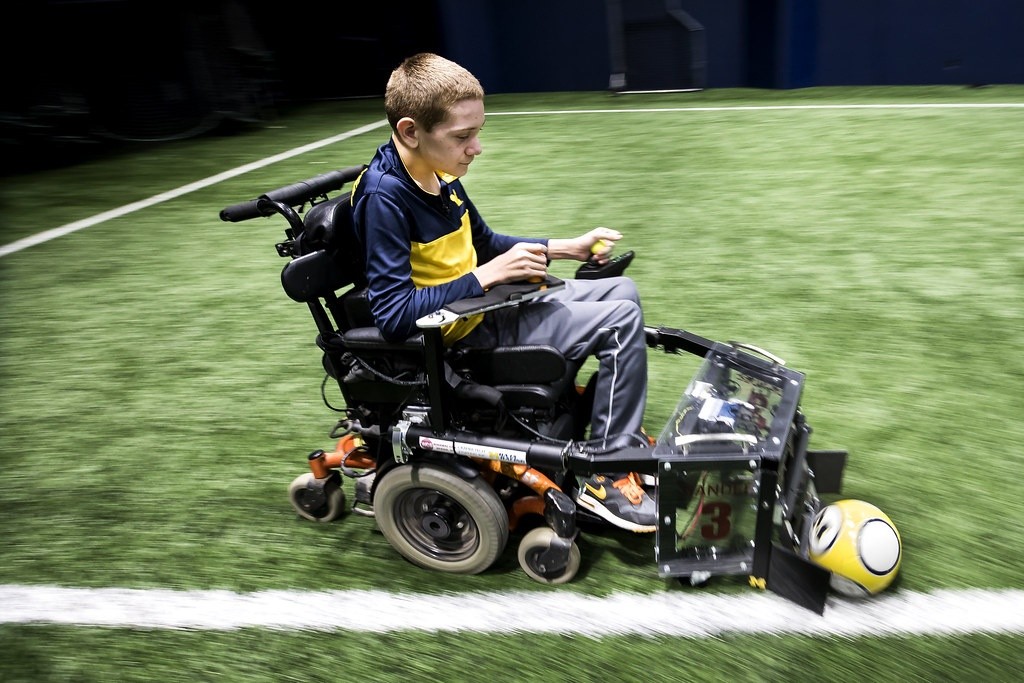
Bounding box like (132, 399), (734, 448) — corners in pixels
(804, 496), (902, 598)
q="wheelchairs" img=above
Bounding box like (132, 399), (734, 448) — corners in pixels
(213, 162), (851, 619)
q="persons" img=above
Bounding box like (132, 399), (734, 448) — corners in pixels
(348, 52), (659, 532)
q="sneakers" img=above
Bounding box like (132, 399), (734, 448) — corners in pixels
(577, 472), (659, 533)
